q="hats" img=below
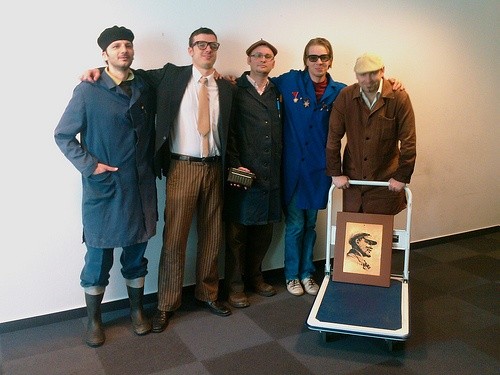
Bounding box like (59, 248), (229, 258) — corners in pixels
(354, 52), (384, 74)
(97, 26), (134, 51)
(246, 38), (277, 56)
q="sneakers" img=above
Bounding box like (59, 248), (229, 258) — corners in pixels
(229, 284), (249, 307)
(285, 279), (304, 296)
(301, 278), (319, 296)
(250, 275), (276, 297)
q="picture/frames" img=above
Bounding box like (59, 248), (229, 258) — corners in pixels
(332, 212), (394, 288)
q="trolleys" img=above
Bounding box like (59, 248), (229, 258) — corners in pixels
(306, 179), (413, 354)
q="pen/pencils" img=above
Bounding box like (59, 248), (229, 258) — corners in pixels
(276, 98), (281, 118)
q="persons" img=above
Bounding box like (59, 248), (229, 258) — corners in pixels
(80, 28), (253, 332)
(223, 37), (405, 296)
(223, 40), (287, 308)
(54, 26), (223, 345)
(326, 53), (417, 216)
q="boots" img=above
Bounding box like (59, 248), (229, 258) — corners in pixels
(85, 286), (105, 346)
(125, 276), (151, 334)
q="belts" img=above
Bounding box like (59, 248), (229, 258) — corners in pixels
(170, 152), (222, 162)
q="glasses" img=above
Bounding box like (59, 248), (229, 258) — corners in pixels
(250, 53), (274, 62)
(192, 41), (220, 51)
(306, 54), (330, 62)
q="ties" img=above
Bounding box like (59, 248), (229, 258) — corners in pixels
(197, 77), (210, 157)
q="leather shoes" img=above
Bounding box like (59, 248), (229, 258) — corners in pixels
(194, 298), (232, 317)
(151, 309), (175, 333)
